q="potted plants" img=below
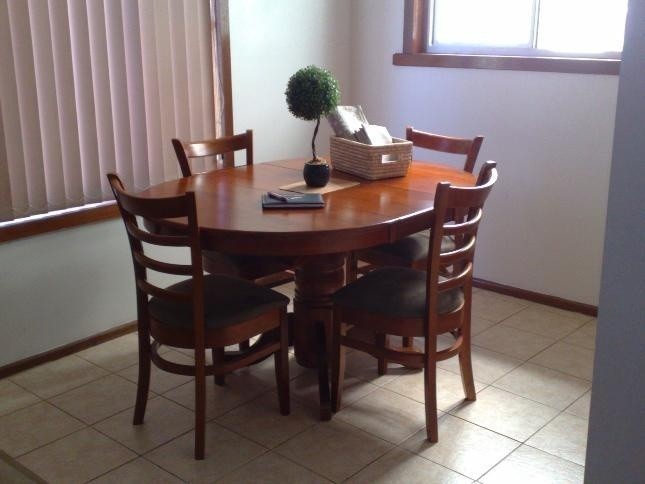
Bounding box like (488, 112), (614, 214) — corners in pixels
(283, 64), (342, 192)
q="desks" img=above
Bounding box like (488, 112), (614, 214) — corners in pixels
(132, 153), (479, 424)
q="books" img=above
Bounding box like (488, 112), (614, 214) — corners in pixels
(328, 104), (397, 162)
(262, 191), (325, 209)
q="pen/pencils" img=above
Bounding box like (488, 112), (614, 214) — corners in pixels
(267, 191), (289, 202)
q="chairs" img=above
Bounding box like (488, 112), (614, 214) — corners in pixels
(104, 170), (294, 462)
(171, 128), (293, 372)
(345, 126), (487, 349)
(330, 158), (501, 442)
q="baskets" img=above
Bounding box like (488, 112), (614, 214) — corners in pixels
(330, 134), (413, 180)
